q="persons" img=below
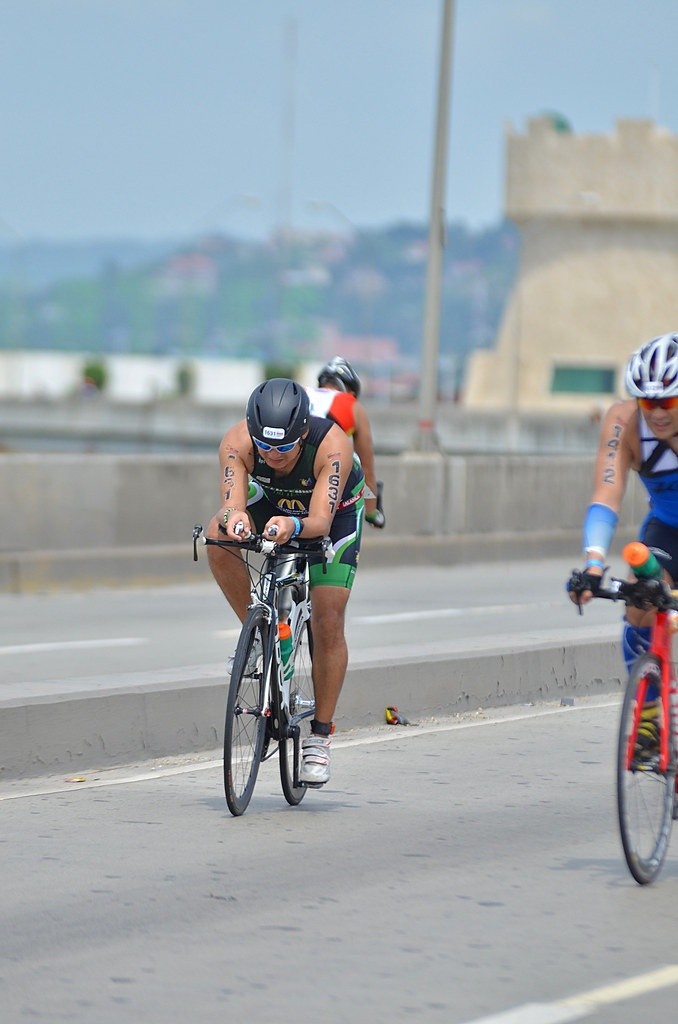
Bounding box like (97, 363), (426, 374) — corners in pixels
(293, 356), (386, 530)
(202, 377), (365, 785)
(566, 331), (677, 817)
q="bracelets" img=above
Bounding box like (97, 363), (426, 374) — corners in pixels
(287, 516), (300, 539)
(580, 558), (609, 570)
(295, 517), (304, 537)
(223, 508), (239, 527)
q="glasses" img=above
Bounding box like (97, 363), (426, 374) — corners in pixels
(639, 398), (677, 409)
(252, 434), (301, 453)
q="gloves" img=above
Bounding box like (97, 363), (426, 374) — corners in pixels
(365, 510), (385, 527)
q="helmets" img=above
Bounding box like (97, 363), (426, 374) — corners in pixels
(248, 379), (311, 445)
(317, 357), (360, 399)
(626, 332), (678, 398)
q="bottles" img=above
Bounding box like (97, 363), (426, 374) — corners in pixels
(277, 622), (294, 681)
(621, 541), (663, 585)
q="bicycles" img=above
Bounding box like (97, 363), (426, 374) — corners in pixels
(570, 541), (677, 886)
(365, 478), (384, 527)
(192, 520), (322, 817)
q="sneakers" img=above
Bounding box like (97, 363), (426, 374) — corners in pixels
(228, 630), (264, 676)
(300, 738), (333, 784)
(629, 738), (667, 769)
